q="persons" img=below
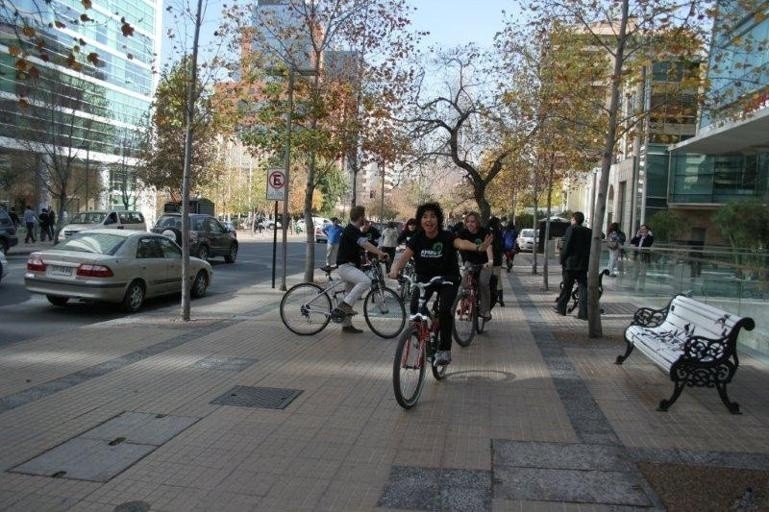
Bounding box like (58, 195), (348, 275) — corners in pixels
(322, 202), (519, 367)
(553, 212), (605, 320)
(606, 222), (626, 278)
(623, 224), (654, 292)
(63, 209), (68, 221)
(0, 203), (56, 243)
(254, 216), (263, 233)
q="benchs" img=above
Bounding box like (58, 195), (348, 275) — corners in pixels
(615, 293), (755, 415)
(559, 269), (610, 314)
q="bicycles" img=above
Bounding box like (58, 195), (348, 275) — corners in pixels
(280, 249), (511, 408)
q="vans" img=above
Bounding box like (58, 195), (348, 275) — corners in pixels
(57, 211), (146, 243)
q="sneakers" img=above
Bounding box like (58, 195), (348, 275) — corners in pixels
(550, 305), (560, 313)
(483, 311), (492, 321)
(341, 326), (363, 334)
(433, 351), (454, 365)
(337, 301), (358, 315)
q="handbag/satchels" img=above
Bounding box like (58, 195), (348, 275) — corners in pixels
(605, 240), (619, 249)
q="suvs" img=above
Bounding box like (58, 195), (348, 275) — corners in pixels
(150, 212), (238, 264)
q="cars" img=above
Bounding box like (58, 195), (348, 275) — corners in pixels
(0, 205), (18, 282)
(24, 229), (213, 313)
(515, 228), (540, 251)
(296, 217), (334, 243)
(244, 217), (282, 230)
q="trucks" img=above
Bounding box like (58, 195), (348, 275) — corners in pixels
(164, 200), (215, 216)
